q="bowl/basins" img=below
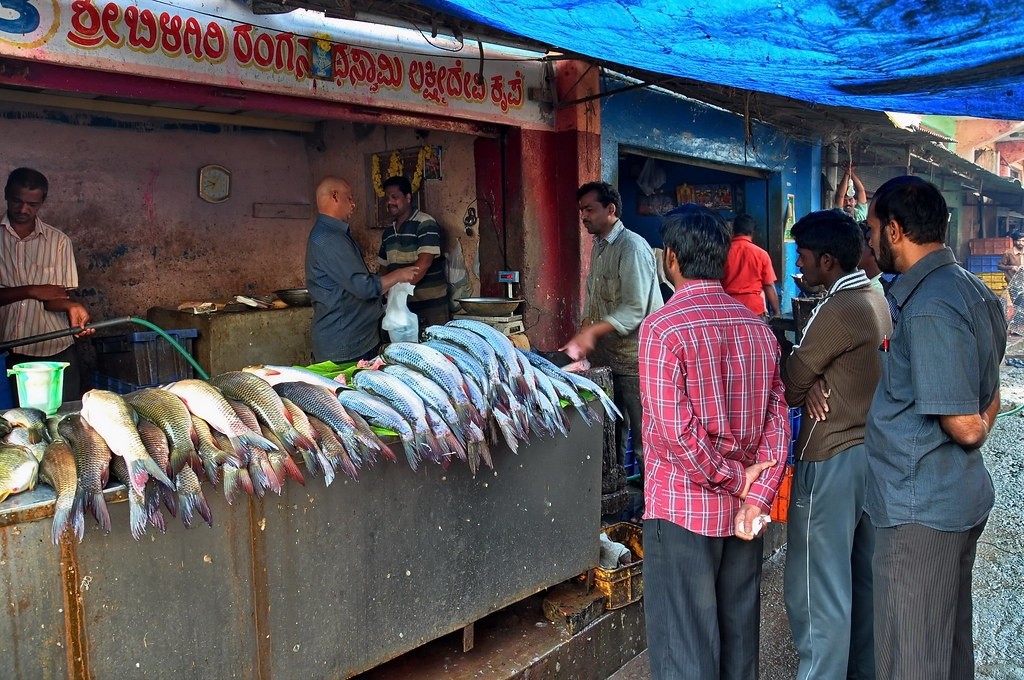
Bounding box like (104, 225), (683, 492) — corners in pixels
(790, 273), (826, 294)
(271, 288), (312, 307)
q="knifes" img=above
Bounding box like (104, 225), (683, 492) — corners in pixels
(537, 350), (571, 369)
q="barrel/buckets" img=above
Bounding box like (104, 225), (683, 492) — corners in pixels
(6, 361), (71, 415)
(0, 350), (18, 410)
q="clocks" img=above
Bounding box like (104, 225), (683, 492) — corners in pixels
(199, 164), (231, 203)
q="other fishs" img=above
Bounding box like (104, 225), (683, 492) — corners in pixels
(0, 320), (625, 545)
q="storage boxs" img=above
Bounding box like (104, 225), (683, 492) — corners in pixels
(580, 519), (644, 610)
(768, 404), (803, 524)
(89, 327), (200, 393)
(966, 236), (1014, 319)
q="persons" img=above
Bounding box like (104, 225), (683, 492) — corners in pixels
(997, 229), (1024, 336)
(637, 201), (793, 679)
(722, 213), (781, 333)
(377, 176), (450, 329)
(864, 174), (1007, 679)
(0, 167), (95, 409)
(557, 180), (664, 492)
(781, 209), (893, 680)
(304, 175), (420, 364)
(857, 220), (898, 329)
(836, 162), (869, 224)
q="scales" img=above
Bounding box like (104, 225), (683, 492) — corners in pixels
(452, 271), (526, 337)
